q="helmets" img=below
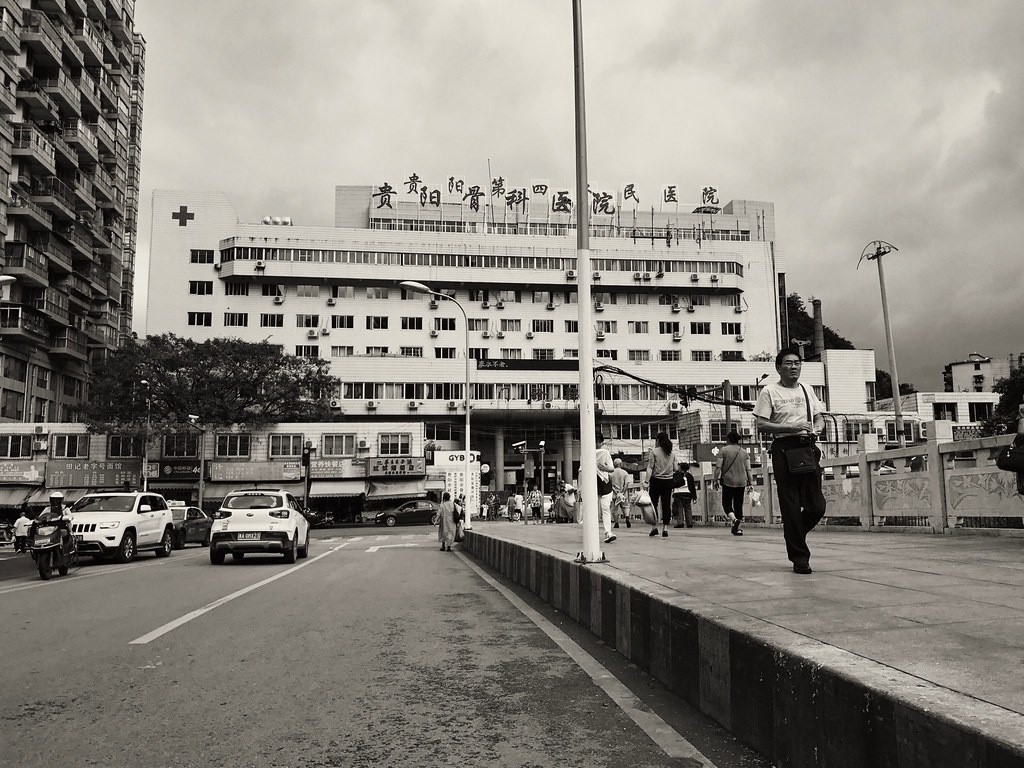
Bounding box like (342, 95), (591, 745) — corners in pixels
(50, 492), (63, 498)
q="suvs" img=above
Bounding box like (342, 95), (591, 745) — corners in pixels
(210, 488), (310, 564)
(69, 490), (174, 563)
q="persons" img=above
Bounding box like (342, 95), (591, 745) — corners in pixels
(610, 458), (631, 528)
(482, 491), (500, 520)
(643, 432), (697, 537)
(751, 347), (826, 575)
(713, 432), (754, 536)
(434, 492), (465, 551)
(577, 431), (617, 542)
(554, 480), (576, 523)
(26, 492), (74, 567)
(13, 512), (31, 554)
(505, 490), (523, 522)
(527, 485), (542, 524)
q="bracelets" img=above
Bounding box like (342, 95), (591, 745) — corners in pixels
(715, 480), (717, 482)
(645, 481), (648, 484)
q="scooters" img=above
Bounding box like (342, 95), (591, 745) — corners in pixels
(23, 502), (78, 580)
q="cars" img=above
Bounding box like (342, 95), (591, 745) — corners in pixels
(167, 500), (213, 550)
(374, 500), (440, 527)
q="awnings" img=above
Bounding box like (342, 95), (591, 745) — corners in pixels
(368, 480), (427, 500)
(309, 481), (366, 497)
(149, 482), (198, 489)
(0, 488), (33, 508)
(203, 484), (256, 502)
(27, 489), (88, 507)
(257, 481), (305, 501)
(425, 481), (445, 491)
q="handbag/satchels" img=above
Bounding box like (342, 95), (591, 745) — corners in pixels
(785, 446), (815, 475)
(615, 491), (625, 506)
(674, 474), (690, 494)
(672, 501), (678, 516)
(455, 526), (465, 542)
(453, 502), (459, 523)
(11, 536), (16, 543)
(997, 445), (1024, 472)
(631, 488), (658, 525)
(672, 472), (685, 488)
(573, 494), (583, 524)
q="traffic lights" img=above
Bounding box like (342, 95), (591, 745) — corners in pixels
(303, 447), (310, 466)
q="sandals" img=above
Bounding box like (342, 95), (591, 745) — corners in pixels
(731, 519), (743, 535)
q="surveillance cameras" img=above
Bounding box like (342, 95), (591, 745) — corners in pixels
(188, 414), (199, 420)
(512, 441), (526, 446)
(539, 441), (545, 447)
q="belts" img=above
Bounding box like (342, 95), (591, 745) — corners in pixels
(778, 437), (813, 444)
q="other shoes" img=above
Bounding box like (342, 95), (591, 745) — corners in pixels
(674, 523), (693, 528)
(626, 518), (631, 528)
(16, 549), (21, 554)
(662, 531), (668, 537)
(604, 533), (616, 543)
(614, 523), (619, 528)
(649, 528), (658, 536)
(794, 563), (812, 574)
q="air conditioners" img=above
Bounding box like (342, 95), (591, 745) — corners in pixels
(918, 421), (929, 439)
(29, 422), (50, 451)
(255, 260), (266, 270)
(408, 400), (418, 408)
(673, 332), (682, 340)
(871, 426), (887, 436)
(214, 263), (222, 271)
(670, 400), (684, 412)
(741, 427), (754, 437)
(321, 328), (330, 335)
(526, 332), (534, 338)
(367, 401), (378, 408)
(430, 330), (438, 336)
(330, 401), (341, 408)
(482, 331), (490, 337)
(736, 335), (746, 341)
(447, 400), (458, 408)
(303, 438), (318, 449)
(543, 402), (552, 409)
(497, 331), (505, 338)
(596, 329), (605, 340)
(307, 329), (318, 338)
(357, 439), (370, 448)
(326, 269), (743, 314)
(273, 295), (283, 305)
(575, 403), (580, 410)
(463, 399), (474, 407)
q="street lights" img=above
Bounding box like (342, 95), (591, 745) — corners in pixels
(867, 241), (906, 448)
(400, 281), (471, 530)
(141, 380), (152, 491)
